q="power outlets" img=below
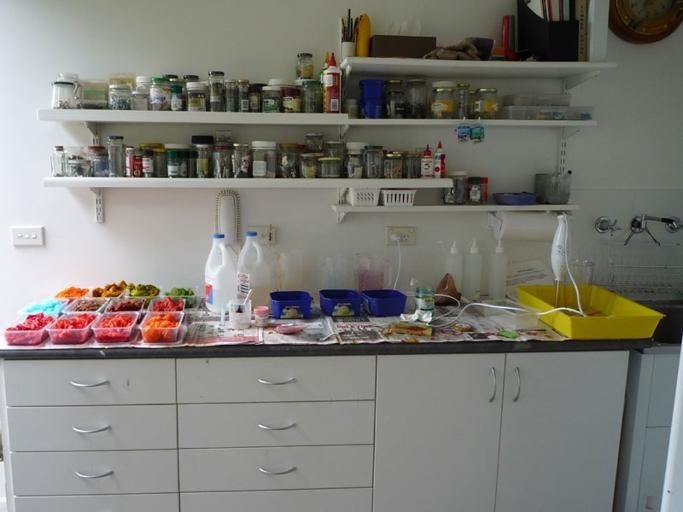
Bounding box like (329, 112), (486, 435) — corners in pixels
(381, 224), (416, 247)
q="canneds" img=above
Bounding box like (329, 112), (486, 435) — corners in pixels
(415, 287), (435, 311)
(298, 52), (314, 79)
(386, 77), (499, 118)
(86, 132), (420, 177)
(444, 171), (488, 206)
(108, 71), (323, 112)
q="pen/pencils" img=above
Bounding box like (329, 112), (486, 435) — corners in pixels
(341, 10), (358, 41)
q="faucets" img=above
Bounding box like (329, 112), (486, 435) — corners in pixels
(623, 212), (680, 250)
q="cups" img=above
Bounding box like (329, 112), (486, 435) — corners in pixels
(534, 174), (570, 205)
(49, 81), (79, 109)
(357, 79), (388, 118)
(340, 42), (356, 59)
(229, 299), (251, 328)
(563, 260), (594, 313)
(414, 288), (433, 317)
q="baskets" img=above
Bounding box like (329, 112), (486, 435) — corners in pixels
(381, 189), (417, 207)
(345, 188), (380, 206)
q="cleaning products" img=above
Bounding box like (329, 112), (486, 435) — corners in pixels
(448, 238), (510, 304)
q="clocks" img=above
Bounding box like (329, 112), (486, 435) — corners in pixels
(606, 0), (683, 47)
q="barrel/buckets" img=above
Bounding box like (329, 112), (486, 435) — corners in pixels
(203, 233), (236, 315)
(237, 229), (273, 316)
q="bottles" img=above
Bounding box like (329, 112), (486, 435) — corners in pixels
(253, 306), (269, 327)
(48, 133), (445, 179)
(382, 78), (497, 119)
(355, 14), (371, 58)
(444, 170), (488, 205)
(106, 51), (340, 114)
(462, 237), (482, 299)
(446, 240), (461, 290)
(341, 98), (357, 119)
(488, 239), (505, 299)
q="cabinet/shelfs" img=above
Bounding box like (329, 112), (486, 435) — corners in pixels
(372, 341), (655, 512)
(0, 345), (179, 512)
(330, 52), (620, 226)
(175, 344), (376, 512)
(35, 105), (459, 193)
(612, 342), (682, 512)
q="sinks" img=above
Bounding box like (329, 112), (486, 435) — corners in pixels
(636, 300), (682, 344)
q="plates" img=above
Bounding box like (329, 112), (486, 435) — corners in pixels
(492, 193), (535, 204)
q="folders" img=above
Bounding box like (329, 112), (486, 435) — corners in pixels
(548, 0), (571, 21)
